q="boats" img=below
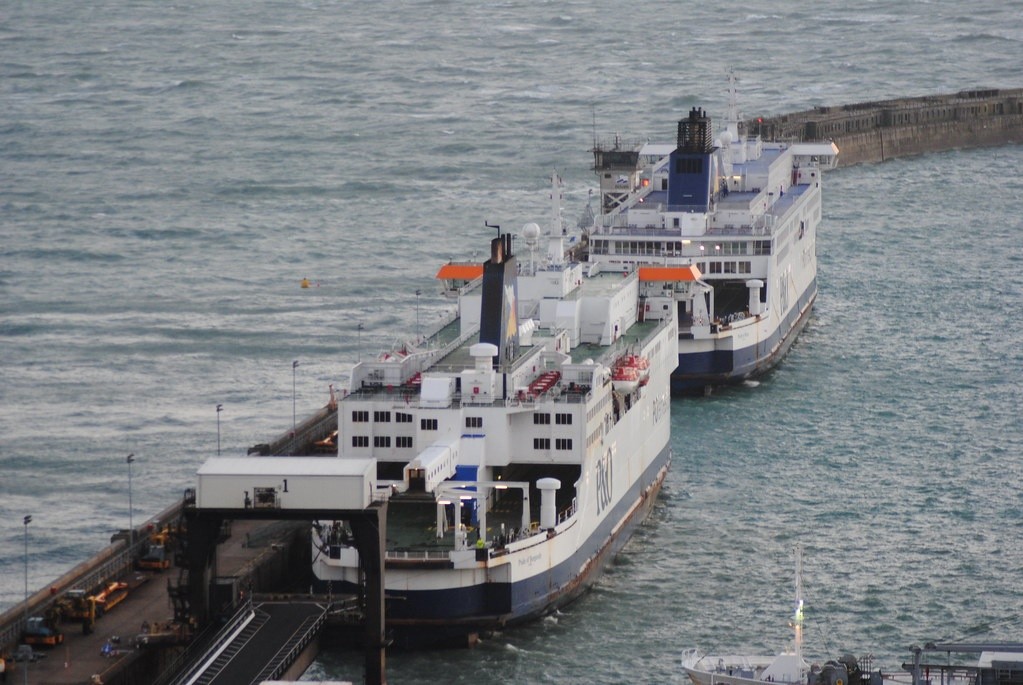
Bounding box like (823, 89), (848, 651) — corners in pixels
(578, 65), (822, 395)
(301, 164), (682, 647)
(680, 538), (913, 685)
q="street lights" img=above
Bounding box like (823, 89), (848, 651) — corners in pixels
(415, 289), (422, 344)
(291, 360), (300, 453)
(24, 514), (32, 639)
(216, 404), (222, 455)
(359, 322), (364, 363)
(127, 453), (135, 573)
